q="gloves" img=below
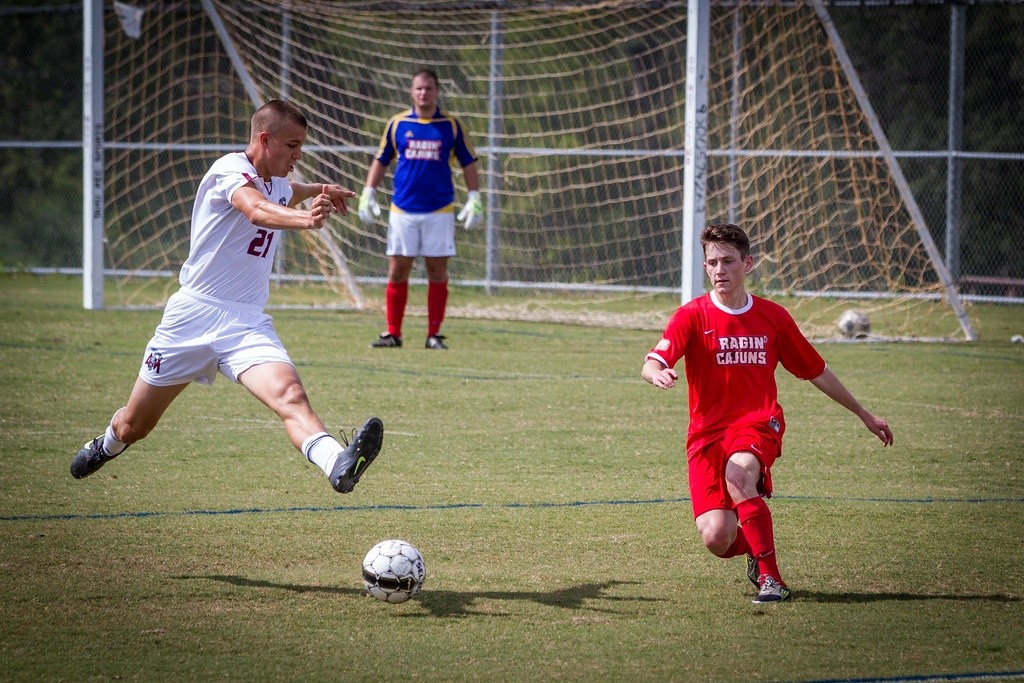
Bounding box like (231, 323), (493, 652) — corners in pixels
(457, 190), (484, 232)
(358, 186), (381, 224)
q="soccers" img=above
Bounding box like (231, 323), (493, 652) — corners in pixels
(835, 308), (870, 339)
(362, 540), (427, 604)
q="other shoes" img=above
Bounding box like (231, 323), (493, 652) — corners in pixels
(426, 334), (447, 349)
(371, 332), (402, 347)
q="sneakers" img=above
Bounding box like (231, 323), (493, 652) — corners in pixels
(746, 555), (760, 590)
(70, 434), (130, 479)
(327, 417), (384, 494)
(758, 576), (790, 601)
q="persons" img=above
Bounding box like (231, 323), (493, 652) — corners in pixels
(640, 223), (892, 603)
(70, 100), (386, 495)
(356, 68), (486, 350)
